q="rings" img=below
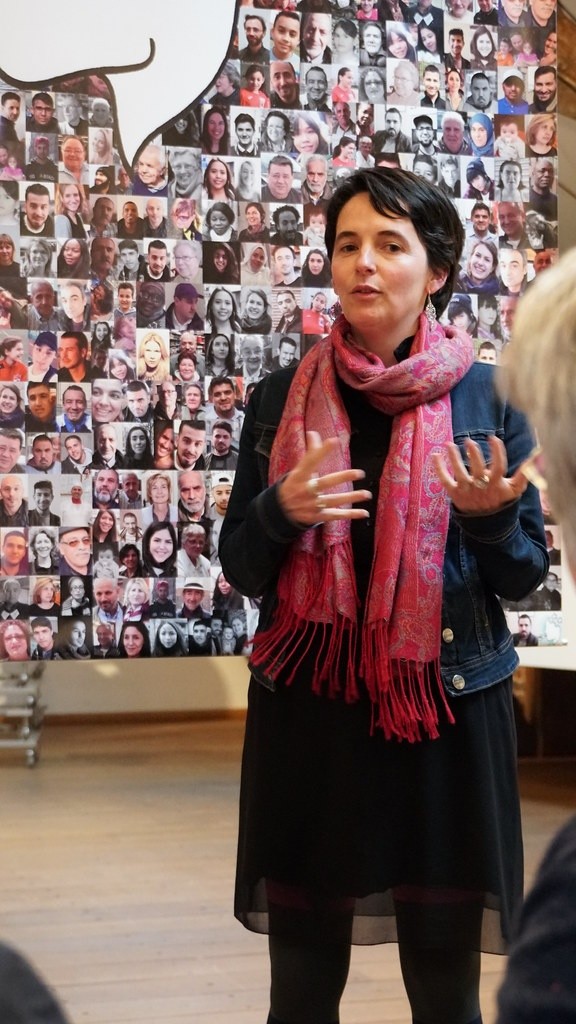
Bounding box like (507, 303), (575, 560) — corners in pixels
(317, 495), (326, 509)
(474, 476), (488, 488)
(308, 477), (323, 494)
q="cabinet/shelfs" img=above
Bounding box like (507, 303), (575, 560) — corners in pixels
(0, 660), (48, 768)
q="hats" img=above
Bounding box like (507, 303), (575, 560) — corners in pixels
(178, 578), (213, 593)
(138, 282), (165, 298)
(174, 283), (204, 300)
(450, 294), (472, 311)
(212, 473), (233, 487)
(413, 115), (434, 128)
(35, 136), (49, 148)
(501, 70), (524, 84)
(467, 157), (485, 173)
(157, 581), (169, 589)
(71, 477), (83, 492)
(34, 332), (57, 352)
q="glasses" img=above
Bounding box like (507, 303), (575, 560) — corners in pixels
(364, 80), (383, 86)
(70, 584), (85, 591)
(393, 74), (415, 83)
(176, 211), (196, 221)
(60, 538), (91, 547)
(33, 105), (53, 112)
(139, 290), (165, 303)
(4, 634), (27, 640)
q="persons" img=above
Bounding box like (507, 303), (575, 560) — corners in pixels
(109, 354), (134, 379)
(56, 385), (92, 432)
(272, 245), (301, 286)
(206, 287), (241, 333)
(218, 166), (548, 1024)
(241, 288), (272, 334)
(124, 426), (154, 468)
(275, 290), (302, 333)
(173, 239), (203, 284)
(137, 279), (166, 328)
(0, 0), (561, 662)
(137, 334), (170, 381)
(61, 435), (92, 474)
(59, 331), (90, 381)
(57, 282), (89, 332)
(241, 244), (271, 286)
(202, 202), (237, 241)
(495, 249), (576, 1024)
